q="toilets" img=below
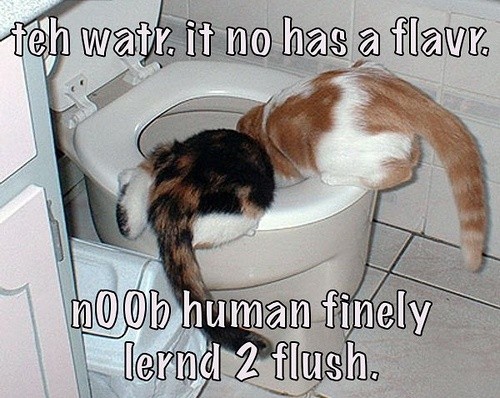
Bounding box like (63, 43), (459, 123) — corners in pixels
(36, 0), (378, 397)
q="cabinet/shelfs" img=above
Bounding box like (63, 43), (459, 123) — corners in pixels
(0, 14), (94, 397)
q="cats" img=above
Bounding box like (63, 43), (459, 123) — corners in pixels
(116, 128), (275, 359)
(236, 59), (487, 272)
